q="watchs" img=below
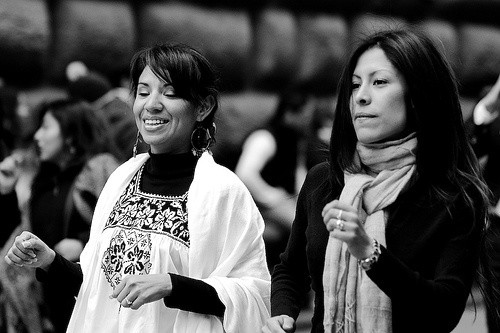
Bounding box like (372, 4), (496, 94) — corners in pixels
(358, 238), (382, 271)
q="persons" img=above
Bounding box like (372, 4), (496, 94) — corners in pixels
(259, 18), (488, 333)
(2, 38), (272, 332)
(233, 80), (323, 229)
(466, 67), (500, 333)
(0, 68), (143, 332)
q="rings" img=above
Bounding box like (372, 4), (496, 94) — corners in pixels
(336, 220), (344, 230)
(337, 209), (343, 220)
(124, 298), (133, 305)
